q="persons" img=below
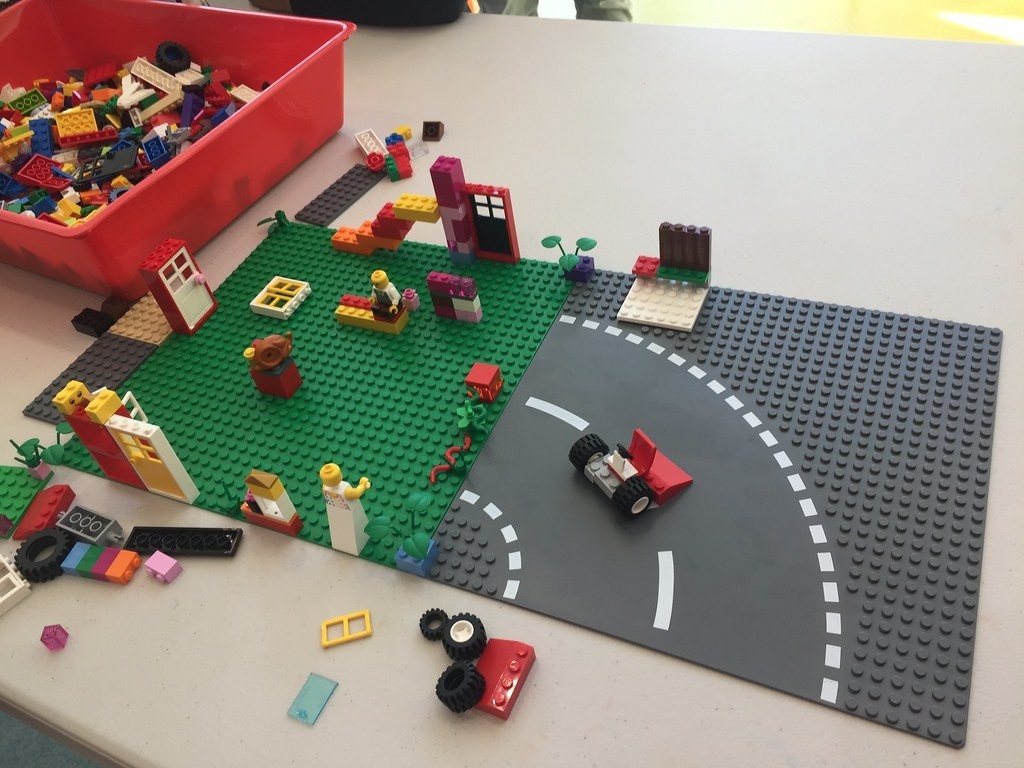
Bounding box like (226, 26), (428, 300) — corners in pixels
(478, 0), (636, 22)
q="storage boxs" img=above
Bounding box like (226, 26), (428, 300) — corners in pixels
(0, 0), (357, 300)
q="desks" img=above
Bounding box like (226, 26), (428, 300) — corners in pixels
(0, 0), (1024, 768)
